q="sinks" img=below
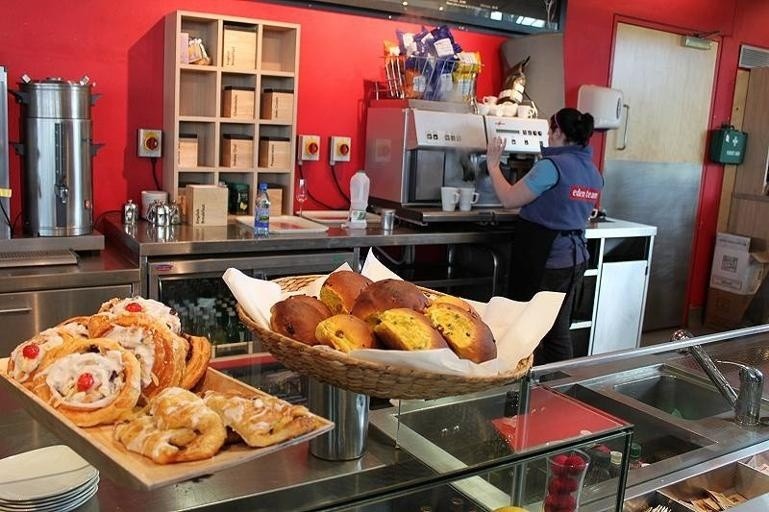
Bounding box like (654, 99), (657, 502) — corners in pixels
(612, 375), (732, 420)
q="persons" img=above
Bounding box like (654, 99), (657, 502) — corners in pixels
(484, 108), (605, 379)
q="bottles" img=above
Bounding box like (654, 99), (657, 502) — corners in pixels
(123, 223), (137, 240)
(123, 200), (136, 225)
(578, 442), (645, 483)
(254, 183), (270, 237)
(346, 168), (370, 229)
(164, 290), (249, 350)
(486, 388), (520, 496)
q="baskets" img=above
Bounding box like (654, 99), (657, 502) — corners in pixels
(236, 271), (536, 400)
(380, 53), (485, 103)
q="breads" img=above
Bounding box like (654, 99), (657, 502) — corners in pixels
(196, 387), (328, 447)
(112, 386), (227, 464)
(270, 271), (497, 365)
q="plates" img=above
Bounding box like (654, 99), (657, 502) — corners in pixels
(0, 446), (103, 512)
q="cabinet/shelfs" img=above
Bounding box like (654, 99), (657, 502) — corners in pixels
(164, 10), (301, 218)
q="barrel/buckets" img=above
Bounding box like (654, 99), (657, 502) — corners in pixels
(349, 170), (370, 232)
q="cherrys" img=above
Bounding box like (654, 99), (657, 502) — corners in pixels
(544, 454), (585, 512)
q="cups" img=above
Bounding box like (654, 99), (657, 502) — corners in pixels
(140, 190), (168, 220)
(475, 94), (535, 118)
(541, 447), (591, 512)
(457, 188), (480, 212)
(379, 208), (401, 231)
(441, 186), (460, 212)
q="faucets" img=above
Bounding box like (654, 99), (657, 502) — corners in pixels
(671, 329), (764, 427)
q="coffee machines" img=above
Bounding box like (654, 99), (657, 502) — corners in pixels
(365, 99), (550, 227)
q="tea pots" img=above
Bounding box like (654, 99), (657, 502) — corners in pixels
(145, 223), (174, 242)
(144, 201), (177, 227)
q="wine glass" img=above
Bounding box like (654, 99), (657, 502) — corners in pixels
(295, 178), (307, 219)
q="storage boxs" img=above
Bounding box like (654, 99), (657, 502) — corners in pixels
(704, 288), (755, 333)
(709, 233), (769, 295)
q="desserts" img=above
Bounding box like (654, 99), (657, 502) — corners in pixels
(7, 294), (213, 429)
